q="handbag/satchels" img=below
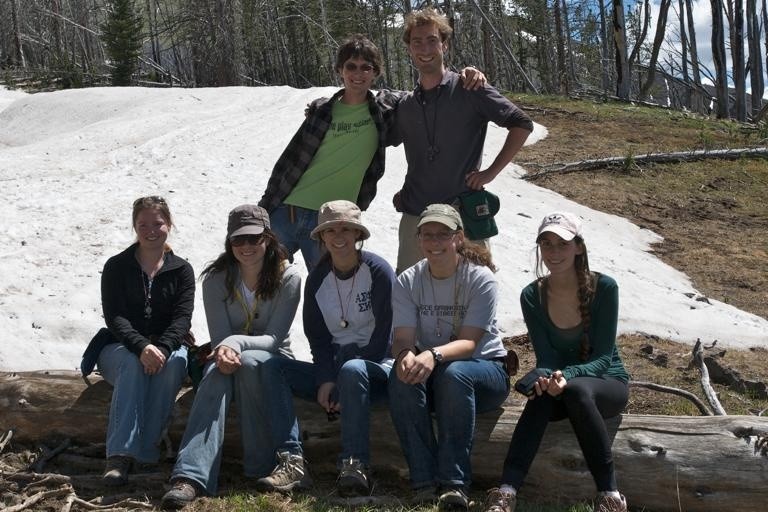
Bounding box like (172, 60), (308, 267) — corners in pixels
(459, 190), (499, 240)
(505, 350), (519, 376)
(80, 328), (112, 376)
(188, 342), (210, 394)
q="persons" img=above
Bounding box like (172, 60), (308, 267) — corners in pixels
(388, 202), (519, 511)
(81, 196), (195, 487)
(483, 213), (629, 512)
(258, 33), (488, 274)
(255, 199), (398, 498)
(304, 7), (533, 276)
(160, 205), (301, 508)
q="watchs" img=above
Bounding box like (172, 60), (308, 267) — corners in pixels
(427, 348), (443, 365)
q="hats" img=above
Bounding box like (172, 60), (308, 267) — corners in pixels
(416, 204), (463, 231)
(309, 200), (370, 241)
(228, 204), (270, 238)
(536, 214), (580, 243)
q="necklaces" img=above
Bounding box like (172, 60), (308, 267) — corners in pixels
(141, 256), (159, 320)
(332, 265), (358, 328)
(420, 86), (440, 162)
(429, 268), (459, 337)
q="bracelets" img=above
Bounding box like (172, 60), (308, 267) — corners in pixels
(398, 348), (416, 356)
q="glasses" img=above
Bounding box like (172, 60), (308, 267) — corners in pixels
(133, 196), (162, 207)
(417, 230), (458, 241)
(230, 237), (264, 246)
(344, 62), (374, 71)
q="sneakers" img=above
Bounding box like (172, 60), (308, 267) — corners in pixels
(256, 451), (314, 491)
(102, 456), (131, 485)
(440, 487), (469, 508)
(594, 493), (628, 512)
(336, 456), (370, 496)
(413, 487), (437, 503)
(483, 486), (516, 512)
(161, 479), (201, 507)
